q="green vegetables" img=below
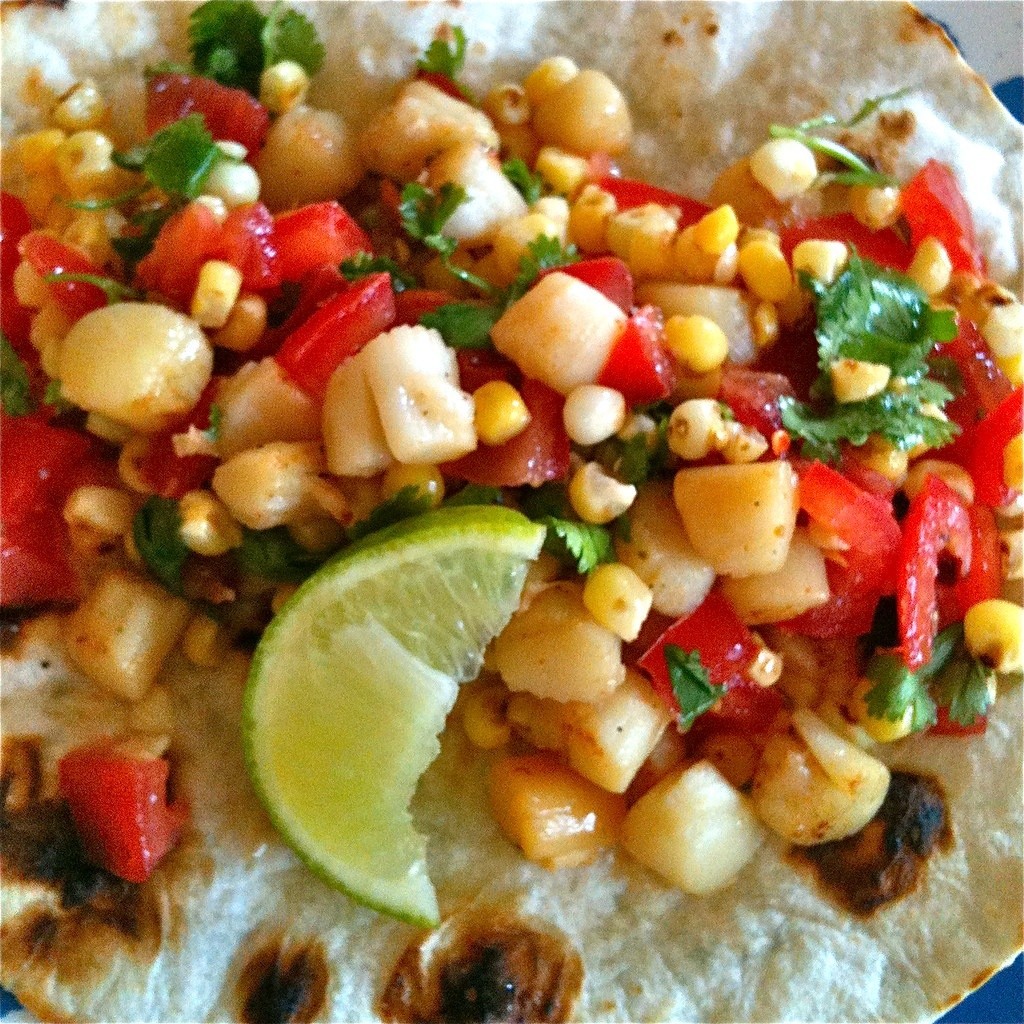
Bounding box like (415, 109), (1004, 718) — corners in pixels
(0, 0), (998, 732)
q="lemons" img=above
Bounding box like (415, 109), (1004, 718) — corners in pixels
(235, 503), (547, 932)
